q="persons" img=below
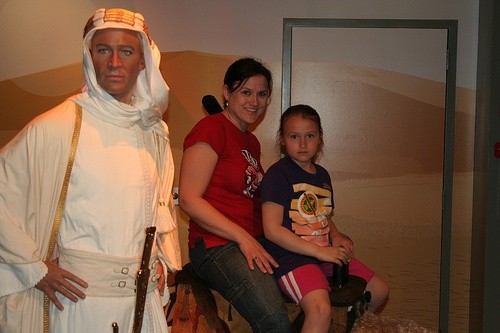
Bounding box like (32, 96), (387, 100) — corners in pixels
(179, 58), (353, 333)
(253, 105), (388, 333)
(0, 8), (182, 332)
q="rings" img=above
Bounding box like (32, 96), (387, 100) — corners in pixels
(254, 256), (259, 260)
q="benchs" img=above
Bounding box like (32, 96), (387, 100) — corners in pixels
(171, 263), (371, 333)
